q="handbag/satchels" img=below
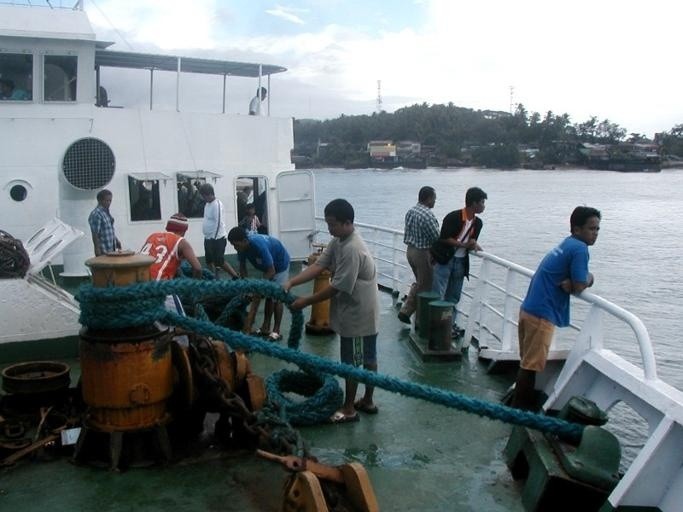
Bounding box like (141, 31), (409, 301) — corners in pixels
(430, 238), (456, 265)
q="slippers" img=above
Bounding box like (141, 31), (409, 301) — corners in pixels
(267, 331), (283, 342)
(354, 395), (378, 414)
(332, 407), (360, 422)
(254, 327), (271, 336)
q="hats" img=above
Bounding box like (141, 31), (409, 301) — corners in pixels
(165, 211), (188, 232)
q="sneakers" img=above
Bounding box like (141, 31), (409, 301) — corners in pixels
(232, 271), (242, 281)
(398, 311), (411, 324)
(450, 323), (466, 337)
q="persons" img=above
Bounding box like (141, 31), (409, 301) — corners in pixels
(88, 190), (122, 259)
(130, 175), (253, 220)
(514, 203), (603, 412)
(397, 185), (440, 326)
(228, 225), (291, 342)
(431, 187), (487, 339)
(199, 183), (239, 280)
(0, 77), (33, 100)
(285, 198), (381, 425)
(237, 203), (263, 235)
(248, 86), (267, 114)
(137, 211), (203, 284)
